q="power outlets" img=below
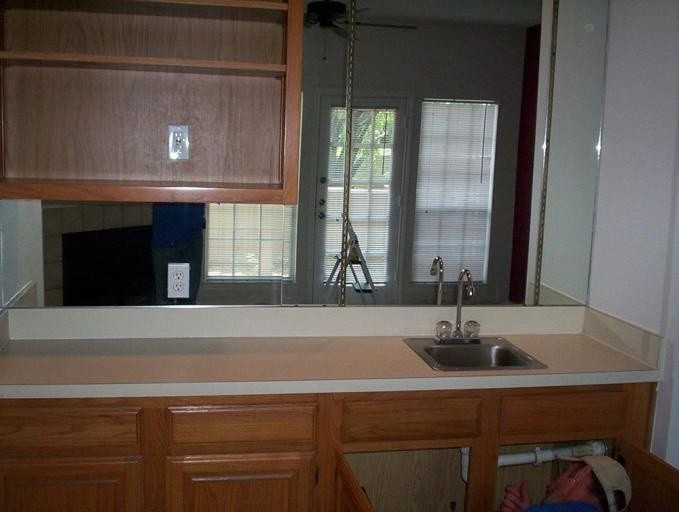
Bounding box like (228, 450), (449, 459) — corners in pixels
(167, 263), (190, 299)
(168, 125), (189, 160)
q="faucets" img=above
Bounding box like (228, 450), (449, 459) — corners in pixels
(431, 256), (444, 303)
(456, 268), (476, 338)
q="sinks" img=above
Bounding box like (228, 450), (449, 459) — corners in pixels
(403, 334), (551, 370)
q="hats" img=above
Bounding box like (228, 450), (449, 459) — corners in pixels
(558, 455), (632, 512)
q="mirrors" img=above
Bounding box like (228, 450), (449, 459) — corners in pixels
(0, 2), (611, 309)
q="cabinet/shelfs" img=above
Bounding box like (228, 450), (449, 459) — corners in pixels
(0, 0), (305, 206)
(331, 382), (679, 512)
(0, 394), (330, 512)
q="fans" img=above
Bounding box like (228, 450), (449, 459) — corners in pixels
(303, 0), (416, 38)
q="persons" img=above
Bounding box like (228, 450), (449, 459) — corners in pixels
(150, 203), (208, 305)
(499, 456), (632, 511)
